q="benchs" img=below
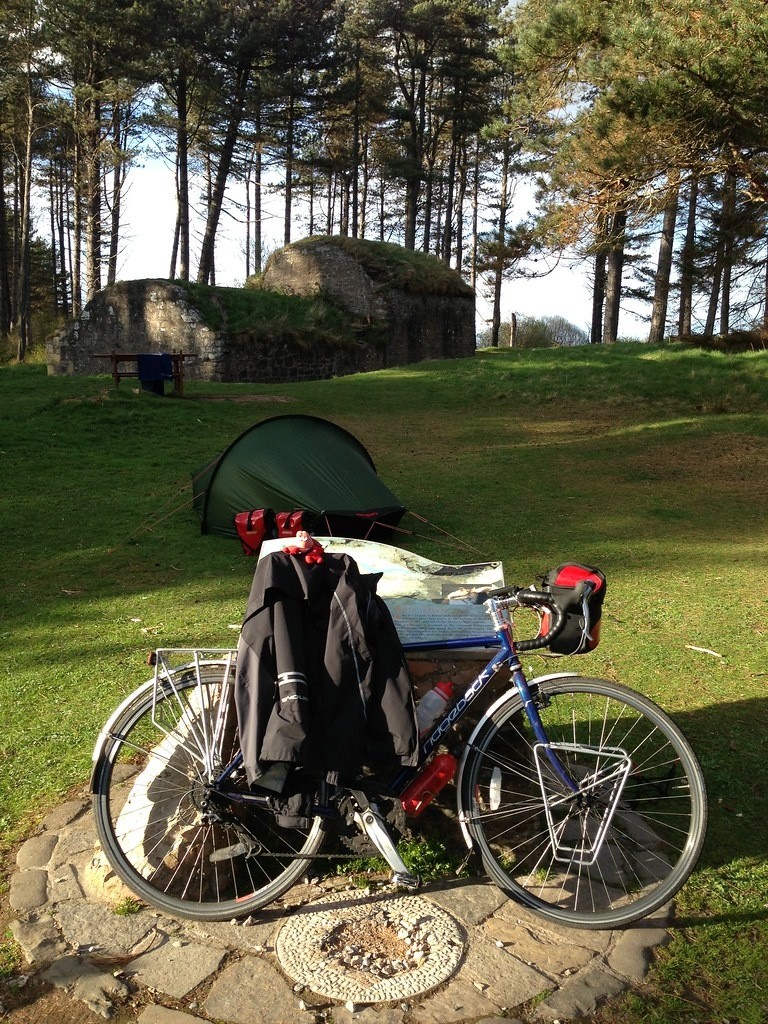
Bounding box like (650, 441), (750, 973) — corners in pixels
(111, 371), (185, 378)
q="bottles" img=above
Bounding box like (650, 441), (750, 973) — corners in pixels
(416, 681), (453, 739)
(401, 750), (460, 817)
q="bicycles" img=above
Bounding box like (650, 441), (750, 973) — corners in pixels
(86, 581), (709, 932)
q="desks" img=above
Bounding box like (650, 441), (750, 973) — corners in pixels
(93, 352), (198, 398)
